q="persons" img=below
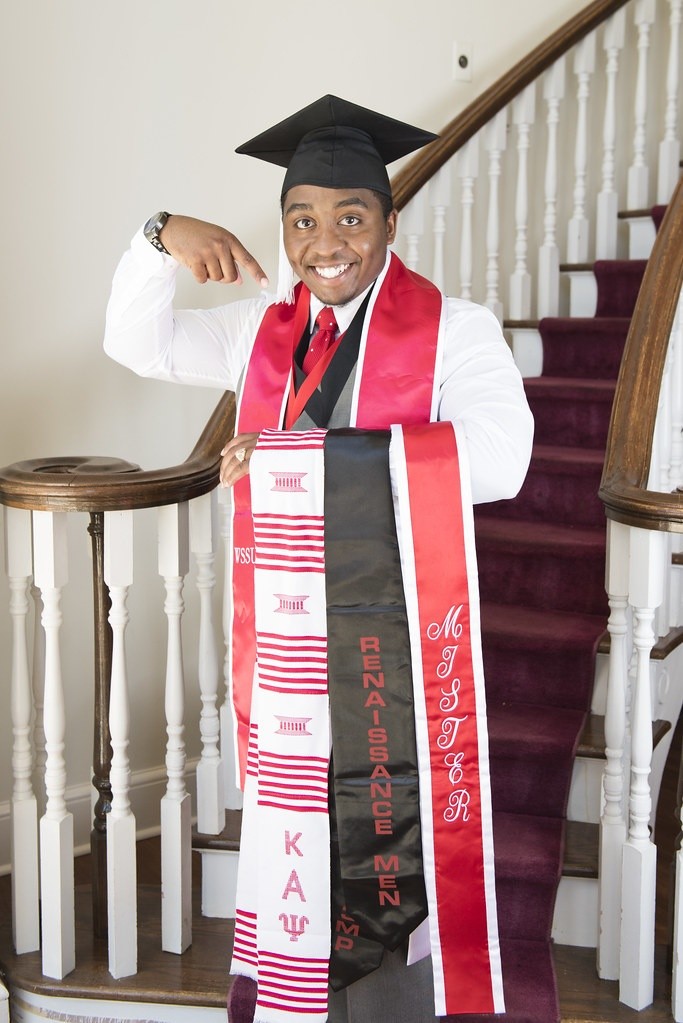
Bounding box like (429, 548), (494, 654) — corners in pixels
(93, 94), (536, 1023)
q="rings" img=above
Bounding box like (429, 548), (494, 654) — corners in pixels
(234, 446), (246, 463)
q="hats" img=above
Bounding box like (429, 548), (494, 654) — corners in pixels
(234, 95), (440, 206)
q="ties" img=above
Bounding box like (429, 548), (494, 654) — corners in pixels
(300, 309), (338, 374)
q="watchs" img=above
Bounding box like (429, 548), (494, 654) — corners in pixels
(142, 208), (172, 262)
